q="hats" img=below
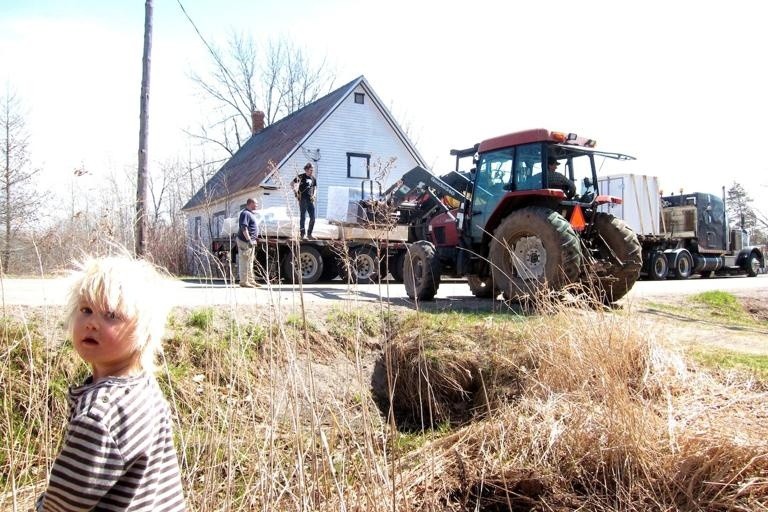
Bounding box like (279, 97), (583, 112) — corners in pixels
(549, 158), (560, 165)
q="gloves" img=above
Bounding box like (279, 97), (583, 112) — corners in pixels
(247, 239), (252, 248)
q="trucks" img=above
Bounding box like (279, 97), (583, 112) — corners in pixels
(211, 190), (764, 284)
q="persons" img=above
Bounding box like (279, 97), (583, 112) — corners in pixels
(289, 162), (317, 241)
(28, 246), (187, 512)
(524, 156), (578, 196)
(236, 198), (262, 288)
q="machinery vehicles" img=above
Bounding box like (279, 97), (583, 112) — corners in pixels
(359, 127), (643, 305)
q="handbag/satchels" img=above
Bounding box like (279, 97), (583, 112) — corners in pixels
(298, 190), (301, 201)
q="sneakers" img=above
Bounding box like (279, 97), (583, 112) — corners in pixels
(301, 235), (317, 240)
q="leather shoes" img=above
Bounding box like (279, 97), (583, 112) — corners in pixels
(240, 282), (261, 288)
